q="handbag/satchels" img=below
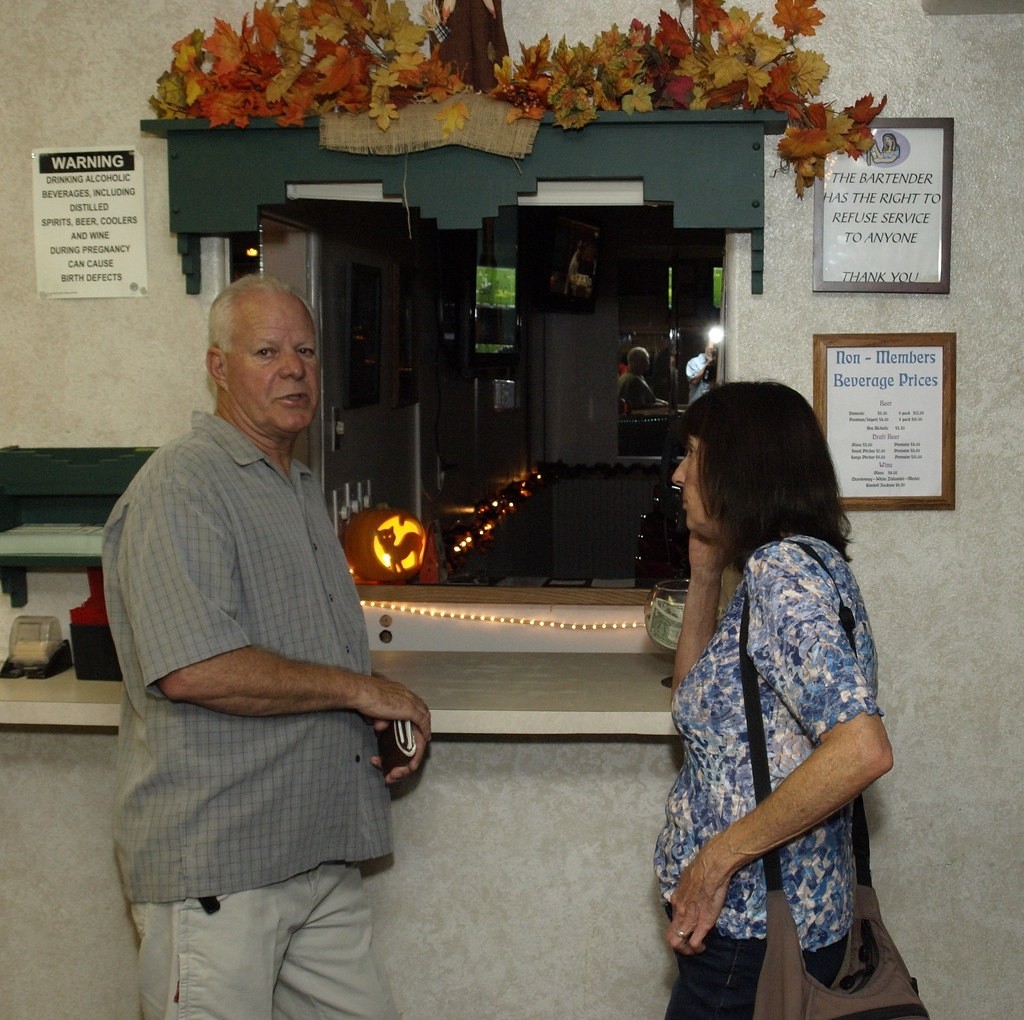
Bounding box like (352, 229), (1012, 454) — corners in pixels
(753, 885), (930, 1019)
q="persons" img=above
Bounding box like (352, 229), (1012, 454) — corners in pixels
(656, 380), (894, 1020)
(618, 343), (720, 408)
(101, 271), (432, 1019)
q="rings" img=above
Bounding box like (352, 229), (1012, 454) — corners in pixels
(676, 930), (685, 937)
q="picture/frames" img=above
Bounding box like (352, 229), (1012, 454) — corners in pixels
(342, 263), (380, 414)
(386, 261), (423, 409)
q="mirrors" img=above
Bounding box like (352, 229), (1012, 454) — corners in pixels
(616, 258), (721, 460)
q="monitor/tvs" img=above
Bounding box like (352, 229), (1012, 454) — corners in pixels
(516, 212), (604, 316)
(443, 255), (521, 368)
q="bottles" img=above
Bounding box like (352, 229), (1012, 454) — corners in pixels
(644, 579), (730, 653)
